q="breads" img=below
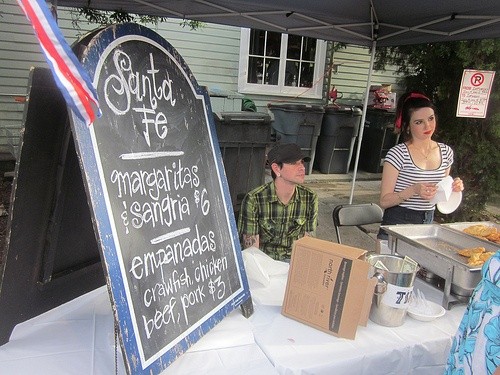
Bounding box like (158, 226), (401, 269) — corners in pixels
(463, 224), (500, 246)
(457, 247), (496, 267)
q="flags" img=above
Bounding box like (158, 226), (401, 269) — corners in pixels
(16, 0), (102, 127)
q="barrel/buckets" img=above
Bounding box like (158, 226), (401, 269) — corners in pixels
(365, 253), (422, 329)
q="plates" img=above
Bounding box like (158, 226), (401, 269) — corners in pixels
(437, 191), (462, 214)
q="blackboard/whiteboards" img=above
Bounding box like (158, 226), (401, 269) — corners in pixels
(0, 21), (255, 375)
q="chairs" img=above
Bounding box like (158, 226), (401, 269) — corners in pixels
(332, 202), (384, 244)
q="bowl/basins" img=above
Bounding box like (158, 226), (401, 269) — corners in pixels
(408, 299), (447, 322)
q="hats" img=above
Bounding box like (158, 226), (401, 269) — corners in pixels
(267, 143), (311, 165)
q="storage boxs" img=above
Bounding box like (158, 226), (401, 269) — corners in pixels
(362, 90), (396, 108)
(281, 236), (378, 341)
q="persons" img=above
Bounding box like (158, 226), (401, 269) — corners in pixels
(442, 250), (500, 375)
(238, 143), (317, 263)
(375, 90), (465, 255)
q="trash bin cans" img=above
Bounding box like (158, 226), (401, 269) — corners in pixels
(315, 103), (363, 174)
(212, 110), (272, 229)
(358, 107), (401, 174)
(267, 103), (325, 176)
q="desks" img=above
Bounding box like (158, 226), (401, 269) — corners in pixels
(0, 264), (472, 375)
